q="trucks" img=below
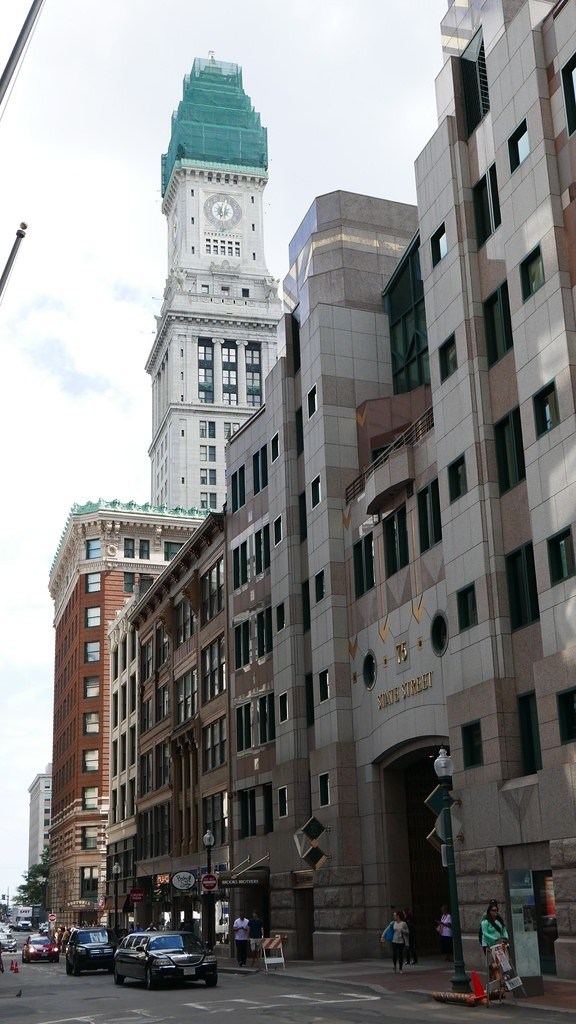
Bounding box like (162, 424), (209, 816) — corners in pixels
(9, 907), (33, 932)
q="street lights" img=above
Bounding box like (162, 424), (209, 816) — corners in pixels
(434, 749), (472, 990)
(202, 829), (215, 952)
(112, 862), (121, 935)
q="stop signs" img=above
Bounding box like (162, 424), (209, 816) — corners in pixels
(49, 914), (56, 922)
(201, 874), (218, 891)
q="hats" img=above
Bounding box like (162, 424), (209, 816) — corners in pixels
(490, 899), (497, 906)
(252, 909), (260, 917)
(404, 907), (411, 916)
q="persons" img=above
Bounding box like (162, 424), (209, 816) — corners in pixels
(54, 925), (71, 956)
(69, 921), (96, 934)
(113, 924), (134, 938)
(145, 923), (157, 932)
(246, 909), (264, 966)
(434, 906), (454, 962)
(232, 913), (250, 967)
(39, 922), (45, 933)
(403, 907), (418, 966)
(381, 911), (410, 974)
(132, 922), (144, 933)
(478, 900), (512, 992)
(160, 918), (194, 932)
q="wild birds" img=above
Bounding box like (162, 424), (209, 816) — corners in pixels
(15, 990), (22, 997)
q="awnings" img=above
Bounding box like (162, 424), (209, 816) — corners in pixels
(103, 895), (130, 913)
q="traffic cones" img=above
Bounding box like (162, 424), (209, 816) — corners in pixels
(470, 970), (488, 1000)
(9, 960), (15, 971)
(12, 962), (20, 973)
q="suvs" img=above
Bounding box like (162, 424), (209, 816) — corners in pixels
(112, 930), (218, 990)
(65, 927), (118, 975)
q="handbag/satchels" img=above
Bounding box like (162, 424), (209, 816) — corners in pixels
(436, 925), (441, 932)
(402, 921), (410, 948)
(384, 921), (394, 942)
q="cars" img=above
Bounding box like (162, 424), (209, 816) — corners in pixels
(38, 922), (50, 936)
(0, 933), (19, 953)
(0, 922), (14, 934)
(21, 934), (61, 963)
